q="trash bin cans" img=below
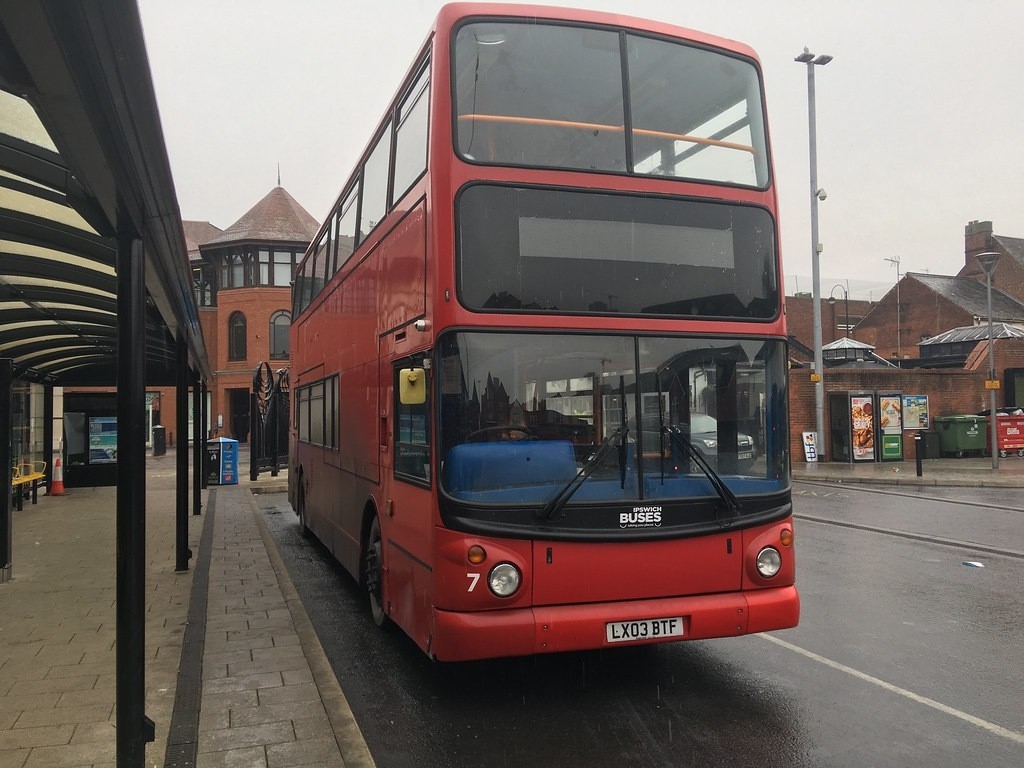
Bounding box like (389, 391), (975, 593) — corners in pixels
(207, 436), (239, 485)
(934, 412), (988, 459)
(981, 406), (1024, 458)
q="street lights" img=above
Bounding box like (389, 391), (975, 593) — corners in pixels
(827, 284), (849, 339)
(793, 46), (833, 463)
(974, 251), (1001, 468)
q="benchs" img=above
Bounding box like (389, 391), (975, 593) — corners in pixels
(12, 460), (46, 510)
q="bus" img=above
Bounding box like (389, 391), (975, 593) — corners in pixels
(284, 9), (802, 661)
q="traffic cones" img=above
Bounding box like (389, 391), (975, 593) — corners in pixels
(50, 459), (66, 496)
